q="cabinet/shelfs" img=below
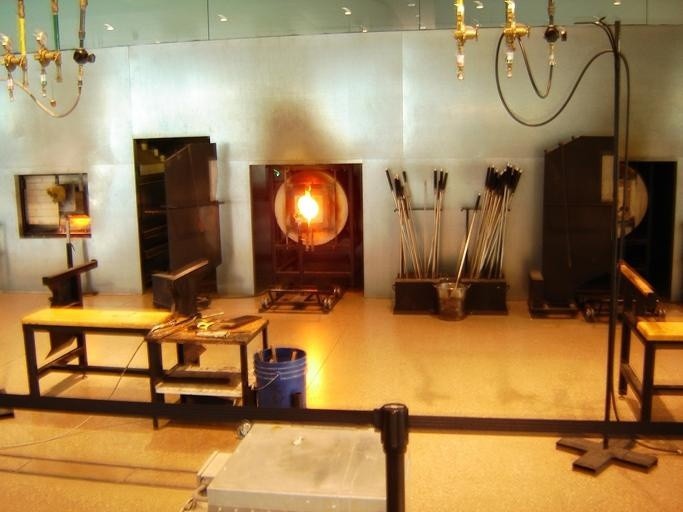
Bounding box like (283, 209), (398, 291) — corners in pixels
(132, 137), (174, 297)
(617, 161), (656, 300)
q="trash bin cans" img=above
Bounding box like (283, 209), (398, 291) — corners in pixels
(256, 349), (305, 410)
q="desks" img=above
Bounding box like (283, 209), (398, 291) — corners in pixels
(21, 307), (271, 430)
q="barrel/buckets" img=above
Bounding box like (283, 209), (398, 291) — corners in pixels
(251, 347), (307, 407)
(432, 282), (472, 321)
